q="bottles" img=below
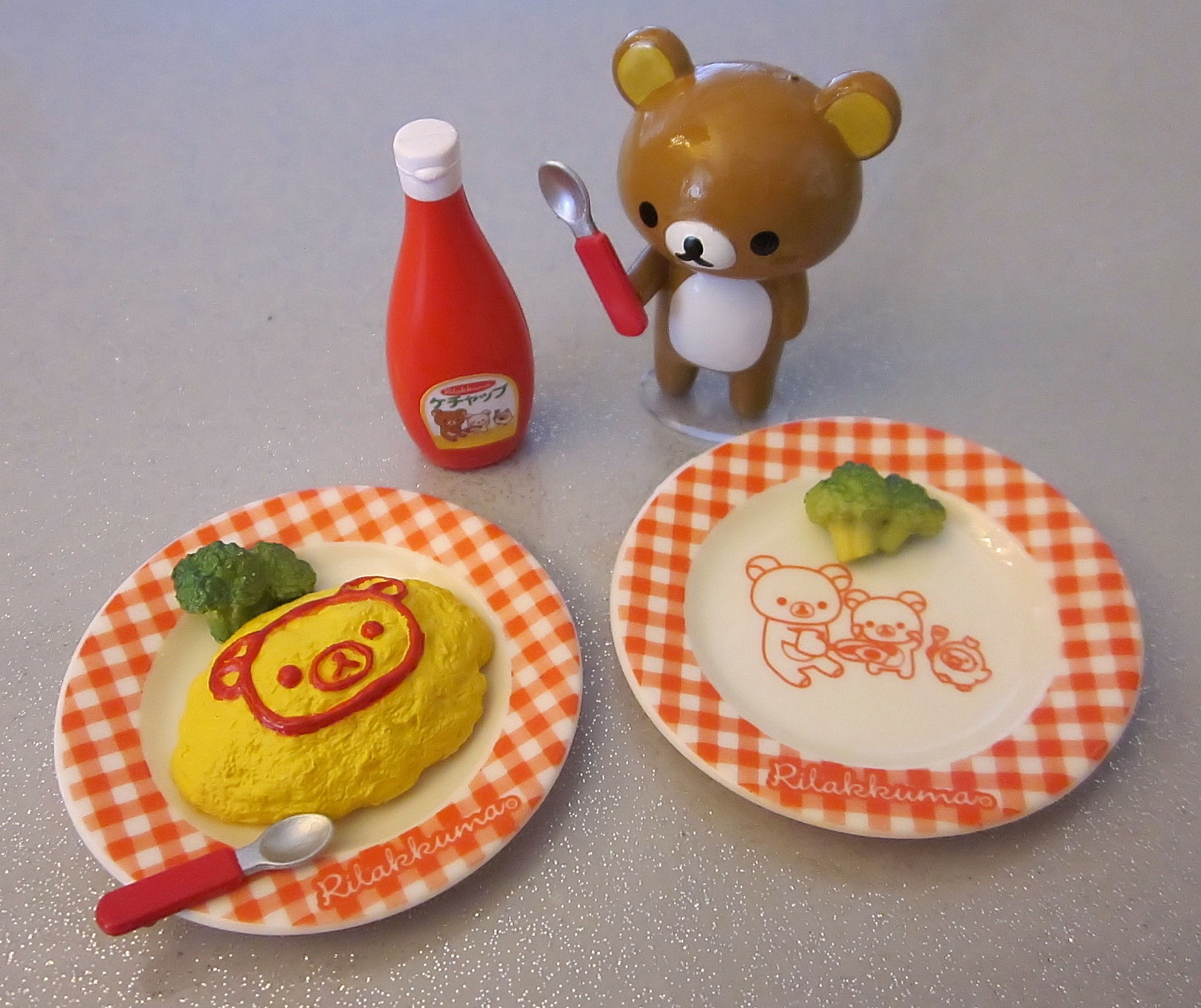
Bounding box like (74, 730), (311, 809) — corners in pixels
(385, 118), (535, 470)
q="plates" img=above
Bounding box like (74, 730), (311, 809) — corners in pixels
(608, 415), (1146, 839)
(48, 484), (583, 936)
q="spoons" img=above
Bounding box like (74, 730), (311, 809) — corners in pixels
(538, 160), (649, 336)
(96, 812), (332, 935)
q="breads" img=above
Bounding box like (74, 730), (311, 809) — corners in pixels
(168, 574), (494, 828)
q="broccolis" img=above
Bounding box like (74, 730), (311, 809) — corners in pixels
(169, 542), (318, 644)
(804, 461), (947, 563)
